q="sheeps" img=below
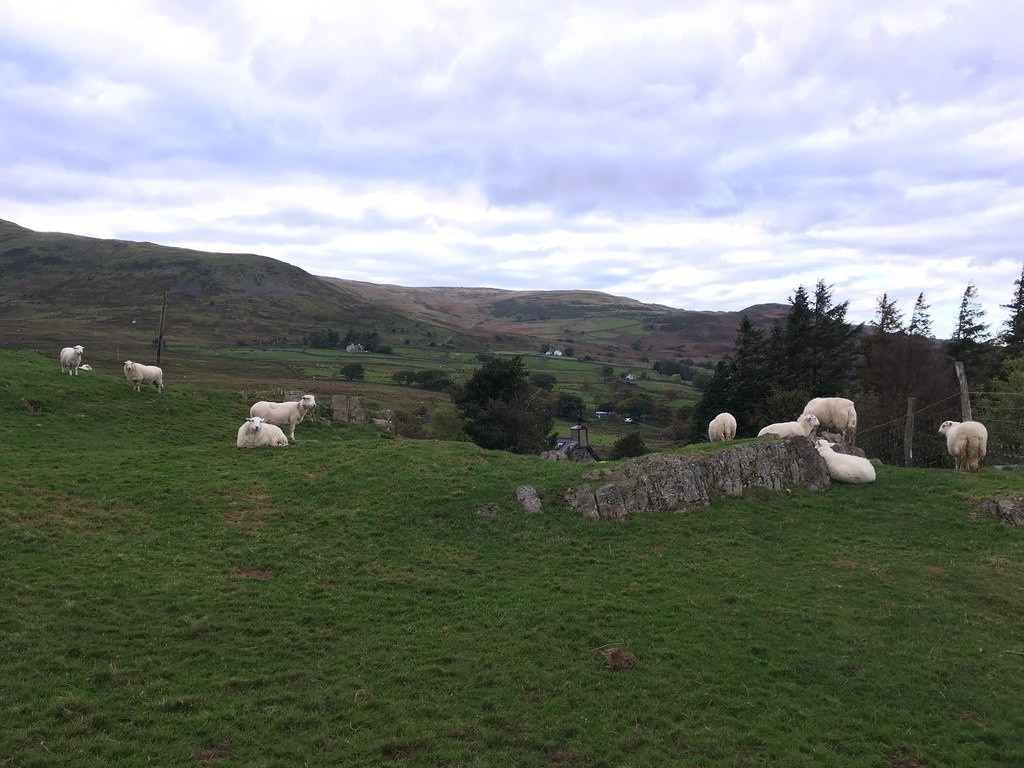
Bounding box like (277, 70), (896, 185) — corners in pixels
(815, 439), (876, 484)
(123, 360), (164, 396)
(249, 394), (316, 439)
(756, 414), (821, 438)
(797, 398), (857, 444)
(939, 421), (987, 472)
(59, 345), (86, 376)
(237, 417), (289, 448)
(708, 412), (736, 442)
